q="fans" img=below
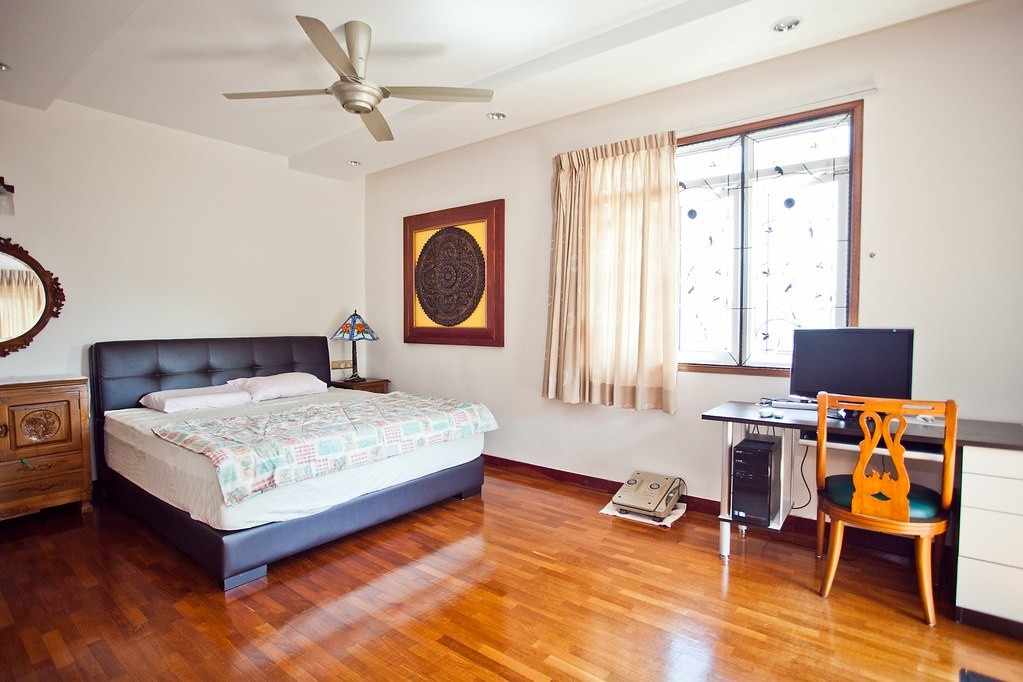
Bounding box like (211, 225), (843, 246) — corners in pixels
(223, 15), (495, 142)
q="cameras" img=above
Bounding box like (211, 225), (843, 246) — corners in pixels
(790, 328), (914, 400)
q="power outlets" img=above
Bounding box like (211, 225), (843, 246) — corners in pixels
(331, 360), (354, 369)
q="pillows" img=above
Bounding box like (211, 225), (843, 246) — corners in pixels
(138, 383), (252, 414)
(226, 371), (327, 403)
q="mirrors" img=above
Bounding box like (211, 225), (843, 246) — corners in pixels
(0, 237), (66, 358)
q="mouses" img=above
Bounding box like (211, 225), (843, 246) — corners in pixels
(916, 414), (936, 423)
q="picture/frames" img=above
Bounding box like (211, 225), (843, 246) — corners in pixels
(402, 198), (505, 348)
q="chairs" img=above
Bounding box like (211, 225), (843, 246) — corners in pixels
(815, 391), (958, 628)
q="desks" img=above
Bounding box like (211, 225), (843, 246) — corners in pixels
(700, 400), (1023, 641)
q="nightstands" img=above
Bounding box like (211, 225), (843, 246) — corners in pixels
(331, 377), (391, 394)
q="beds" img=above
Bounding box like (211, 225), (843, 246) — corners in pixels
(88, 335), (500, 592)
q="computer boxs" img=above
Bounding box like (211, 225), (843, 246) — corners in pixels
(733, 433), (782, 525)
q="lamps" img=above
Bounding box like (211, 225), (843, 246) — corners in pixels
(330, 309), (380, 383)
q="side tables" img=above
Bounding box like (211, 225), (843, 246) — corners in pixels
(0, 374), (95, 523)
